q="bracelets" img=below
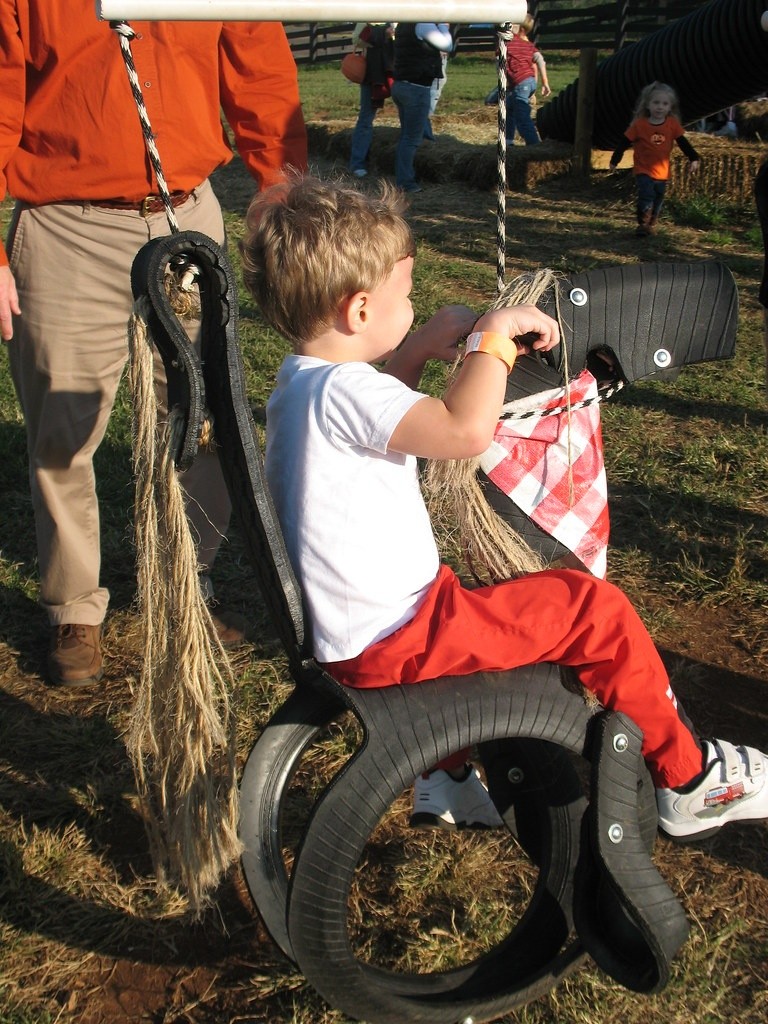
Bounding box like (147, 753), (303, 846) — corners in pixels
(465, 332), (517, 374)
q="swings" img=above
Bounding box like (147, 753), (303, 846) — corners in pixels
(106, 18), (697, 1023)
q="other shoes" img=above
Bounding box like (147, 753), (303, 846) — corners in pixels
(354, 169), (367, 178)
(506, 138), (515, 146)
(406, 184), (422, 193)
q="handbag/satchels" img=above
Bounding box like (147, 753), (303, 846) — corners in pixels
(341, 44), (368, 84)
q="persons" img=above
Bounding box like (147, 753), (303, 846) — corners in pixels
(348, 14), (552, 195)
(609, 83), (699, 235)
(238, 171), (768, 842)
(1, 1), (304, 685)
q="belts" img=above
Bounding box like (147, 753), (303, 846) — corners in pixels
(64, 189), (195, 217)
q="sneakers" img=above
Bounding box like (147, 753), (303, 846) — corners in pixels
(656, 738), (768, 844)
(47, 620), (103, 686)
(197, 604), (255, 647)
